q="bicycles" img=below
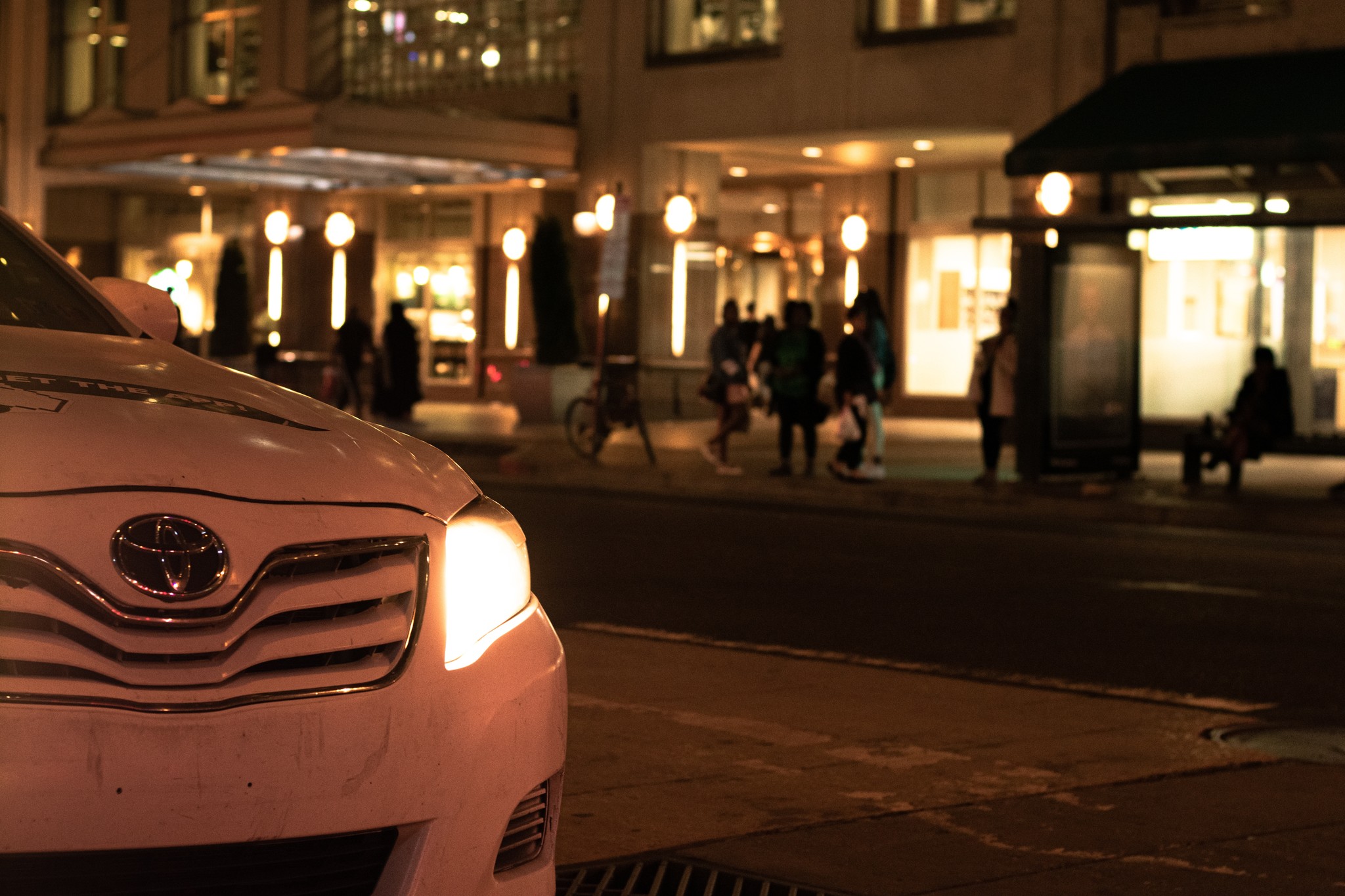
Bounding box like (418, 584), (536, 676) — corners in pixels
(562, 352), (657, 468)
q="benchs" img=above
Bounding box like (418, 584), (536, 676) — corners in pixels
(1112, 414), (1345, 499)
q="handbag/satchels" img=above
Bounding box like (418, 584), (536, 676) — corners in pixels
(727, 383), (753, 403)
(836, 407), (862, 441)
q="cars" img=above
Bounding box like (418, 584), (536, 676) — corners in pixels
(0, 209), (569, 896)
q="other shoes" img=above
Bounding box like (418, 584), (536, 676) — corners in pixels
(825, 461), (867, 484)
(769, 462), (797, 478)
(858, 463), (885, 478)
(699, 445), (742, 476)
(971, 470), (997, 488)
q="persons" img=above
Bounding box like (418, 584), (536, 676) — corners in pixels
(588, 296), (658, 470)
(1203, 348), (1297, 490)
(697, 287), (890, 483)
(320, 303), (424, 427)
(963, 301), (1028, 486)
